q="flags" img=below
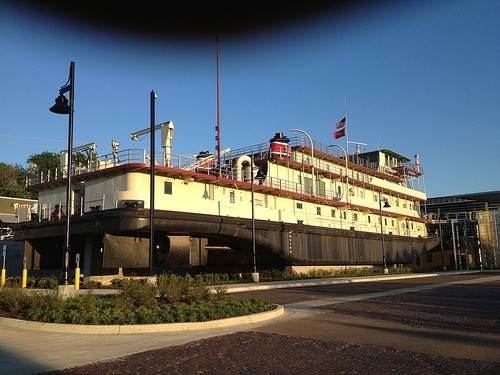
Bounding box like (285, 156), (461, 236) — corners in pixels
(336, 117), (345, 128)
(333, 129), (345, 139)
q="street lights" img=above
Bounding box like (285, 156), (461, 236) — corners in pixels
(379, 198), (392, 276)
(48, 62), (75, 286)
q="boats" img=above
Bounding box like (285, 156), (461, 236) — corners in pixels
(14, 114), (429, 276)
